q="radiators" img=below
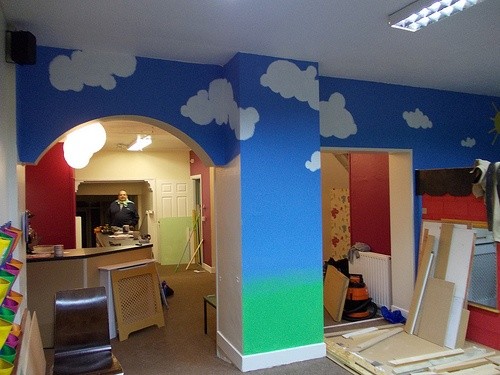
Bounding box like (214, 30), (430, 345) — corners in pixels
(348, 251), (392, 310)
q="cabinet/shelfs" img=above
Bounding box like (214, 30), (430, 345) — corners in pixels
(0, 224), (20, 375)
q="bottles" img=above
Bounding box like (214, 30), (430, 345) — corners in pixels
(100, 223), (114, 234)
(28, 222), (34, 253)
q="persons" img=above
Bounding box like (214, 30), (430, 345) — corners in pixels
(110, 189), (140, 229)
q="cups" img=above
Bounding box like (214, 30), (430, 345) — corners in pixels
(134, 231), (140, 240)
(54, 245), (64, 257)
(123, 225), (129, 233)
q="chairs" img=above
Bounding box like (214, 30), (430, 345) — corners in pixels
(48, 286), (124, 375)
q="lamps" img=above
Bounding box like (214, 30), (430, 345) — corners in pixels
(126, 134), (152, 151)
(386, 0), (478, 32)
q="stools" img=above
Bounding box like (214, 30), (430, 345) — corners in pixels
(202, 295), (216, 334)
